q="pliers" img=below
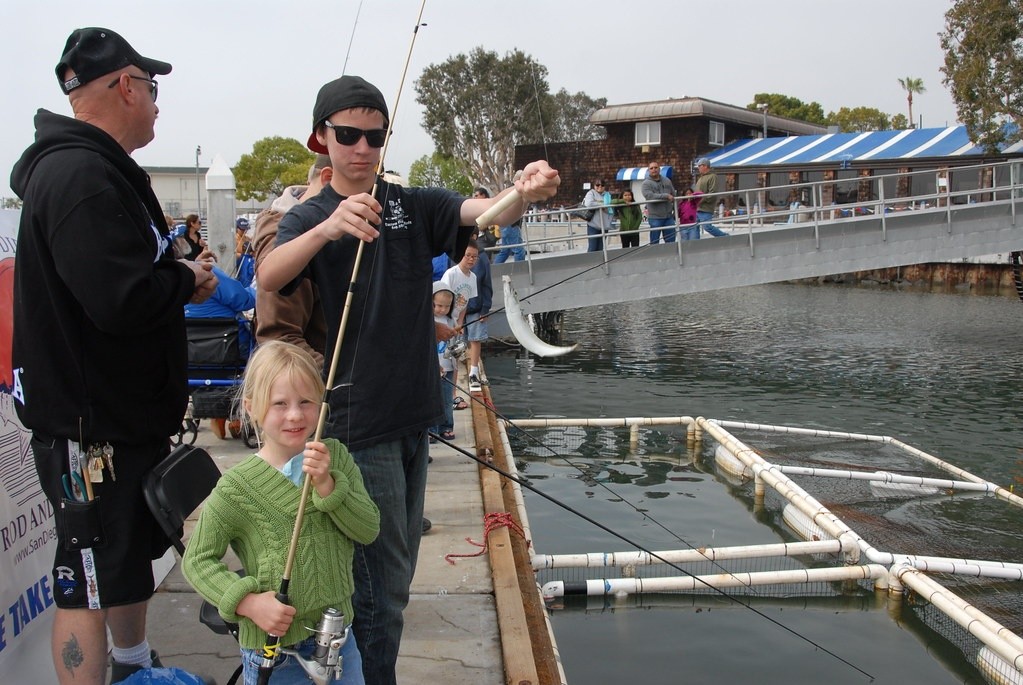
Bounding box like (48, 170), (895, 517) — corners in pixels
(62, 472), (89, 503)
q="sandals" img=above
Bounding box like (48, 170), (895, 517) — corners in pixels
(453, 397), (468, 409)
(440, 429), (454, 439)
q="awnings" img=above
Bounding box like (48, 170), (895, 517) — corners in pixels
(616, 165), (672, 180)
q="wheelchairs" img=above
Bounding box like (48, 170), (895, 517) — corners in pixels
(166, 317), (261, 450)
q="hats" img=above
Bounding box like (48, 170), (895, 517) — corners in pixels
(236, 218), (250, 230)
(695, 158), (710, 166)
(307, 76), (391, 155)
(54, 26), (172, 95)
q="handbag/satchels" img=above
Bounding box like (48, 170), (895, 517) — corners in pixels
(575, 190), (596, 221)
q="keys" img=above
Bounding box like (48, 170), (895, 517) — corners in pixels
(103, 445), (116, 481)
(91, 446), (104, 471)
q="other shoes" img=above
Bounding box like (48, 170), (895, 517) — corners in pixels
(210, 418), (226, 440)
(111, 644), (164, 685)
(421, 517), (429, 535)
(468, 375), (480, 387)
(229, 421), (245, 437)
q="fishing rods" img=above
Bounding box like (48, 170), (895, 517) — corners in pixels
(427, 431), (877, 681)
(454, 211), (726, 331)
(442, 376), (759, 594)
(254, 0), (428, 684)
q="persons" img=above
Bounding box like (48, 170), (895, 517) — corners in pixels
(610, 188), (642, 248)
(677, 187), (703, 240)
(180, 339), (381, 685)
(494, 169), (525, 262)
(257, 76), (560, 685)
(429, 225), (493, 444)
(694, 158), (729, 239)
(161, 213), (258, 437)
(250, 153), (334, 370)
(474, 186), (496, 261)
(8, 27), (219, 685)
(582, 177), (611, 251)
(642, 161), (675, 244)
(544, 202), (558, 211)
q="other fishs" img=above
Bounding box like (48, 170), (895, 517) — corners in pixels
(500, 273), (578, 357)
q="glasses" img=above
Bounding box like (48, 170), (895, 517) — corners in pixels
(109, 74), (158, 102)
(196, 221), (201, 225)
(464, 254), (480, 260)
(325, 119), (394, 148)
(595, 183), (605, 187)
